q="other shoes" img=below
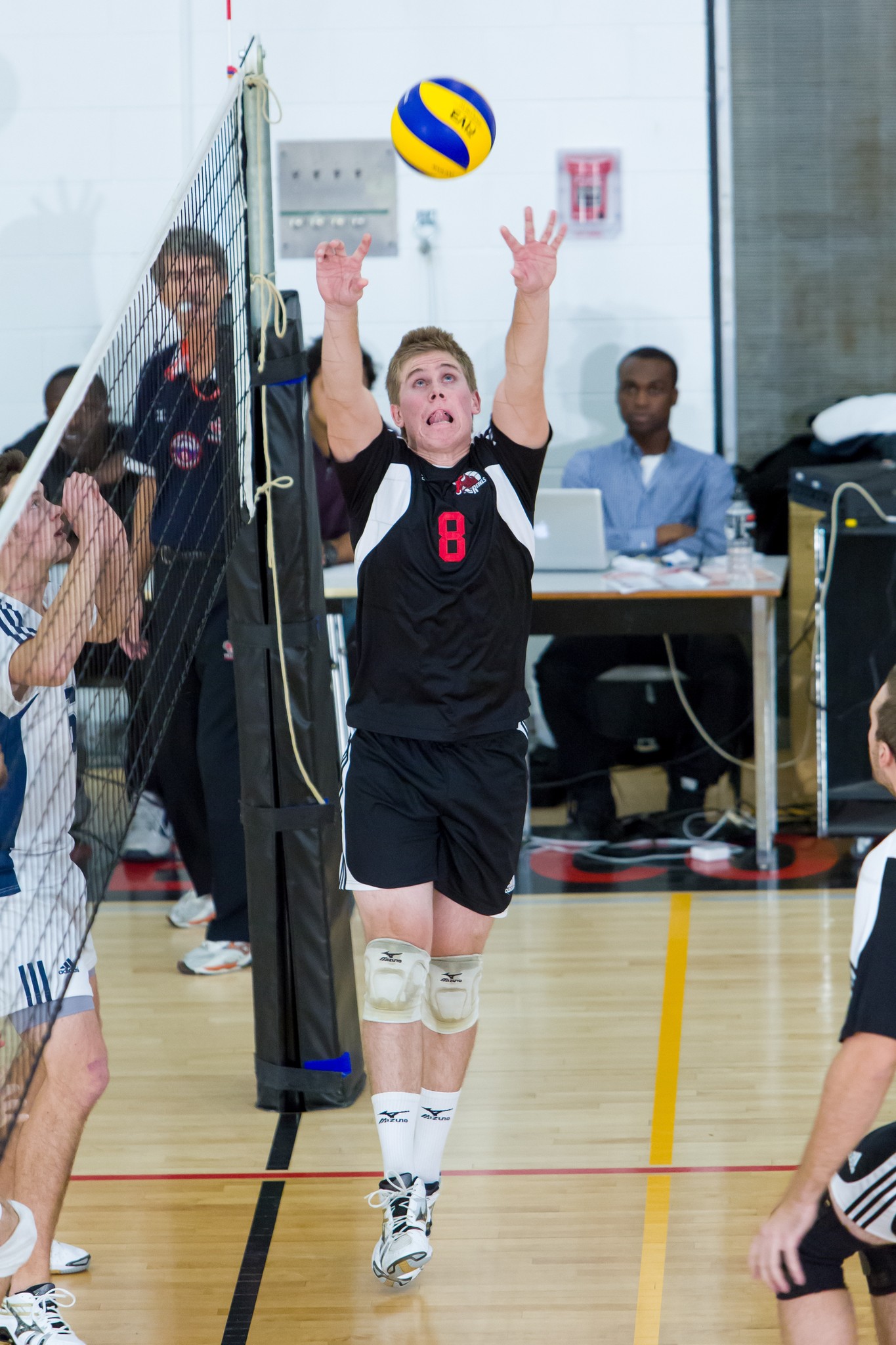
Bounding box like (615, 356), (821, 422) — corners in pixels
(560, 790), (619, 842)
(176, 939), (254, 975)
(119, 788), (175, 861)
(165, 888), (216, 928)
(666, 788), (708, 838)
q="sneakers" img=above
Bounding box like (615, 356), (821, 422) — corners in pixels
(0, 1283), (88, 1345)
(372, 1173), (442, 1288)
(49, 1240), (89, 1274)
(363, 1173), (433, 1279)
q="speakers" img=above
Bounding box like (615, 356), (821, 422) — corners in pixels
(816, 516), (896, 838)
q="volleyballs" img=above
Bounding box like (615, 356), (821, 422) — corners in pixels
(392, 78), (494, 178)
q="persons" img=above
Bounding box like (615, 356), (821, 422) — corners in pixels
(755, 663), (895, 1345)
(0, 202), (749, 1345)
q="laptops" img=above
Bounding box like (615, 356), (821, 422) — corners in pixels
(533, 488), (620, 571)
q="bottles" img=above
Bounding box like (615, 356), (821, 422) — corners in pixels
(725, 486), (756, 580)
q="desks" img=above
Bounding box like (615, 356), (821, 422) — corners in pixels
(529, 555), (789, 870)
